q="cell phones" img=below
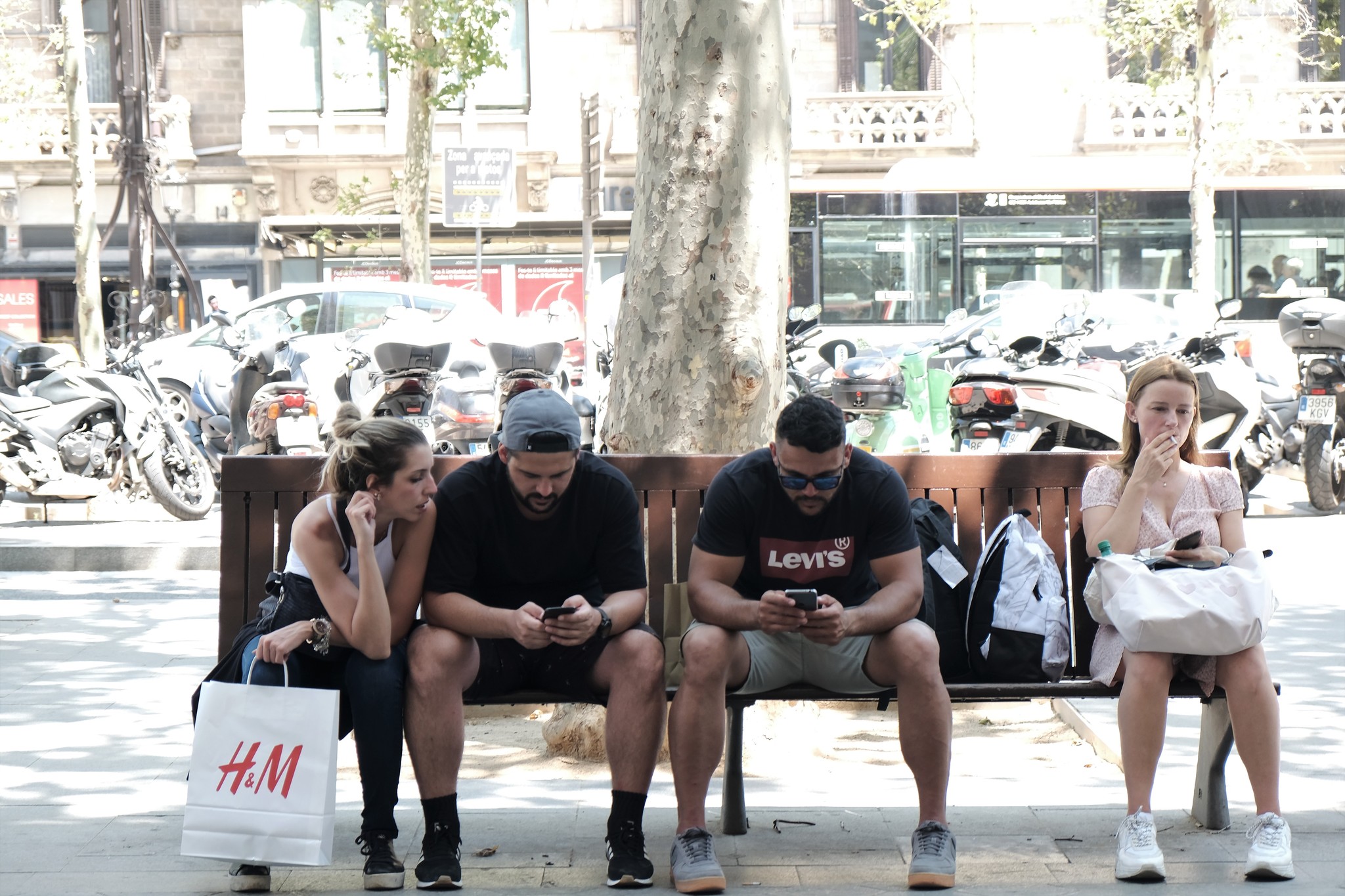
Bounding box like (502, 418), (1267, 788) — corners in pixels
(541, 607), (577, 623)
(1173, 530), (1202, 550)
(785, 589), (818, 612)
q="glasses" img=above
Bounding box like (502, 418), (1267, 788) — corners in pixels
(777, 455), (845, 491)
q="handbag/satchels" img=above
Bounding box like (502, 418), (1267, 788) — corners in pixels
(179, 655), (341, 868)
(1083, 537), (1277, 657)
(255, 497), (351, 635)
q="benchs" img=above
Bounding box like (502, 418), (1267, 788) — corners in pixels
(218, 450), (1281, 835)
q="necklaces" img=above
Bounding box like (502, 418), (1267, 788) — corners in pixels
(1159, 468), (1181, 486)
(377, 526), (387, 545)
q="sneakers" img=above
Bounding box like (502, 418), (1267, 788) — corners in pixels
(1243, 812), (1296, 879)
(907, 819), (957, 890)
(605, 818), (655, 888)
(1114, 805), (1166, 882)
(669, 826), (726, 894)
(354, 833), (407, 891)
(227, 862), (271, 893)
(414, 827), (464, 890)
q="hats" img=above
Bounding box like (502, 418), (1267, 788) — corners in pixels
(497, 389), (582, 452)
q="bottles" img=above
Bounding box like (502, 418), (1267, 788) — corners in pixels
(1097, 540), (1113, 557)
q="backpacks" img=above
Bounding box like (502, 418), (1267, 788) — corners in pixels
(860, 497), (968, 710)
(966, 509), (1070, 686)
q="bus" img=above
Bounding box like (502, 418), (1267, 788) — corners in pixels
(785, 171), (1345, 452)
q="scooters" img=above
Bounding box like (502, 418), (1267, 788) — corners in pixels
(0, 288), (619, 527)
(1280, 290), (1345, 516)
(783, 277), (1286, 511)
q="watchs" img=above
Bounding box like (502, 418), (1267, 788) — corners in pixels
(306, 618), (327, 645)
(594, 606), (612, 639)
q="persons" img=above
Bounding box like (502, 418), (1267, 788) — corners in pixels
(1064, 254), (1095, 291)
(299, 295), (320, 336)
(1078, 354), (1295, 884)
(401, 388), (669, 892)
(204, 295), (229, 325)
(1243, 254), (1341, 298)
(186, 400), (437, 892)
(668, 393), (957, 893)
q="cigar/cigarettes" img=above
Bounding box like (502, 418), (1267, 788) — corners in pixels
(1170, 435), (1178, 445)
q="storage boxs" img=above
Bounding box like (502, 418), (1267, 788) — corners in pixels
(374, 342), (452, 372)
(1278, 298), (1345, 351)
(831, 356), (906, 410)
(1, 341), (60, 389)
(486, 342), (563, 375)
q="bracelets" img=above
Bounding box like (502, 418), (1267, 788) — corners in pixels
(314, 618), (332, 656)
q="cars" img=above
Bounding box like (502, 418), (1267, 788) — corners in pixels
(133, 282), (515, 446)
